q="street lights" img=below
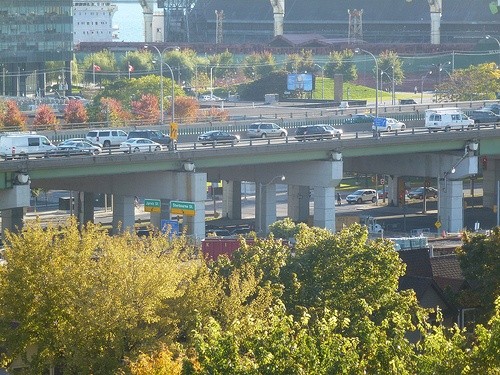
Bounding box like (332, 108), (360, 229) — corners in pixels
(143, 44), (182, 124)
(386, 58), (452, 106)
(353, 47), (377, 131)
(152, 60), (177, 152)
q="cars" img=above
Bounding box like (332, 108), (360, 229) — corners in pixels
(58, 141), (103, 156)
(407, 187), (438, 199)
(119, 139), (163, 152)
(57, 138), (103, 148)
(315, 124), (342, 139)
(372, 117), (406, 132)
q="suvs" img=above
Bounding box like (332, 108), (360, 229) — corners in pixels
(345, 189), (378, 204)
(295, 126), (333, 142)
(127, 130), (177, 151)
(246, 122), (287, 138)
(86, 129), (129, 147)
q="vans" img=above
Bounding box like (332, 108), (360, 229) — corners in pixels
(425, 108), (475, 133)
(197, 130), (241, 147)
(0, 132), (58, 160)
(467, 110), (500, 128)
